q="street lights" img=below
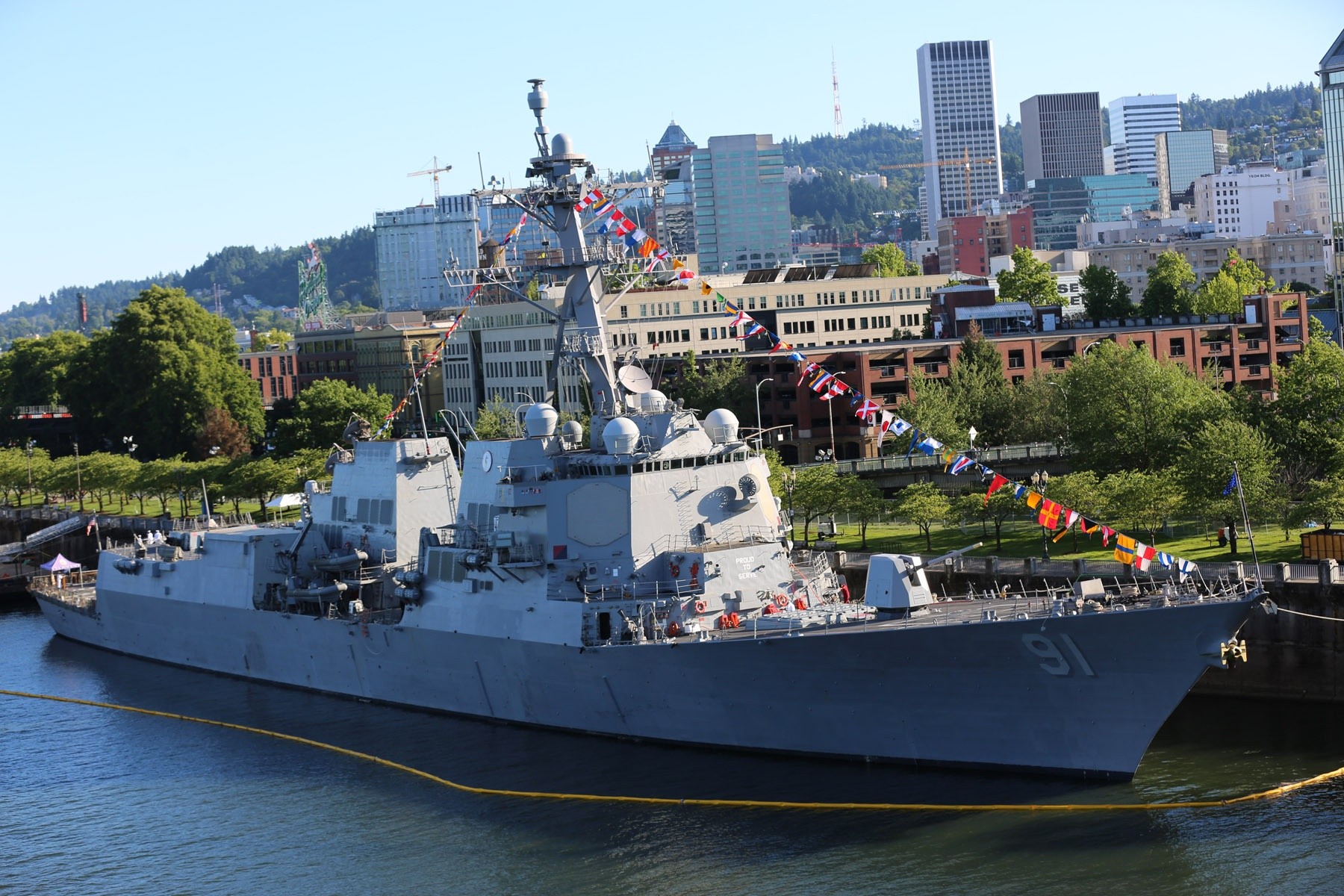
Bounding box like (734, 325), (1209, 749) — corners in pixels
(828, 372), (846, 464)
(1033, 470), (1050, 564)
(1046, 377), (1080, 443)
(781, 470), (796, 549)
(756, 378), (774, 449)
(1081, 341), (1101, 366)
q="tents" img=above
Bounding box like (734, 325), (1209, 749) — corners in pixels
(40, 553), (83, 589)
(264, 493), (308, 522)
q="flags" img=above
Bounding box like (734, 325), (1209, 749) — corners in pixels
(87, 514), (97, 536)
(915, 436), (945, 459)
(1222, 470), (1239, 495)
(941, 446), (1198, 583)
(764, 331), (927, 463)
(377, 283), (482, 436)
(487, 198), (536, 283)
(734, 321), (767, 340)
(569, 186), (754, 327)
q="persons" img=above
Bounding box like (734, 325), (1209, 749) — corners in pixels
(57, 571), (63, 588)
(137, 535), (143, 548)
(839, 583), (850, 604)
(147, 529), (162, 545)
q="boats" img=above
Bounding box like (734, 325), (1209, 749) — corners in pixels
(37, 74), (1268, 784)
(285, 579), (348, 604)
(0, 539), (58, 610)
(306, 547), (368, 574)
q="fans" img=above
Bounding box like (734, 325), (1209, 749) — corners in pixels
(739, 473), (760, 500)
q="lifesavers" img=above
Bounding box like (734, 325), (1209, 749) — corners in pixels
(777, 593), (788, 606)
(719, 613), (729, 630)
(670, 621), (678, 636)
(694, 600), (706, 614)
(794, 597), (807, 610)
(671, 565), (680, 578)
(730, 612), (740, 628)
